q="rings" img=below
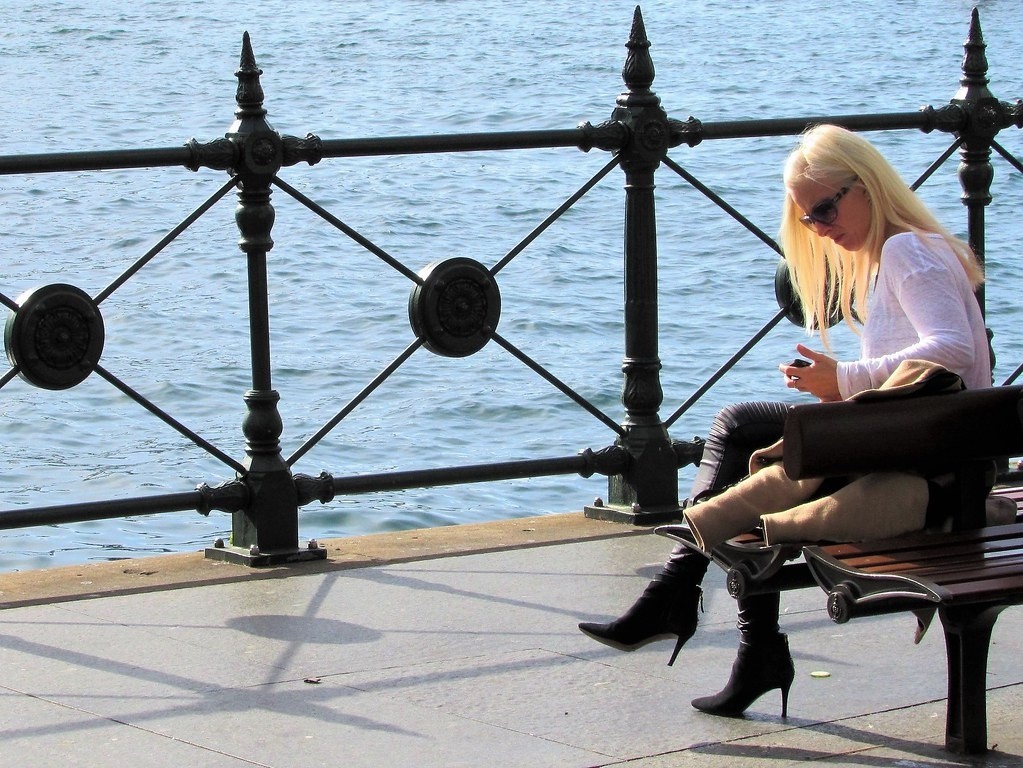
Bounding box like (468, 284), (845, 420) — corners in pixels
(793, 379), (797, 388)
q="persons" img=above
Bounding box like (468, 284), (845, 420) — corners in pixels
(577, 123), (993, 717)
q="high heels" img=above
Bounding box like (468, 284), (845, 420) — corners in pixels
(579, 573), (704, 666)
(693, 633), (795, 716)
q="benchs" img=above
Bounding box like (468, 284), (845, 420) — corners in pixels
(727, 385), (1023, 758)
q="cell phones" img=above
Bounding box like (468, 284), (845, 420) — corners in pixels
(787, 358), (814, 369)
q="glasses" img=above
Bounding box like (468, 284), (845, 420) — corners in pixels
(799, 176), (857, 233)
